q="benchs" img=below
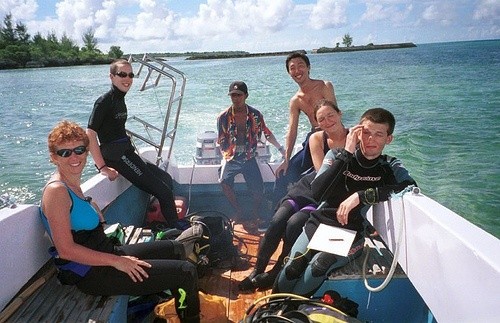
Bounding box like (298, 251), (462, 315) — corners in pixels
(287, 182), (408, 280)
(0, 225), (154, 323)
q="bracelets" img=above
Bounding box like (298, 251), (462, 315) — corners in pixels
(277, 145), (283, 150)
(95, 163), (106, 173)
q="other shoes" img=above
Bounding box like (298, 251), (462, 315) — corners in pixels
(253, 269), (277, 290)
(239, 270), (264, 291)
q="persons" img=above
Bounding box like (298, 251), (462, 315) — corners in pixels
(88, 60), (192, 229)
(273, 52), (338, 203)
(238, 100), (418, 297)
(40, 121), (201, 323)
(217, 81), (286, 222)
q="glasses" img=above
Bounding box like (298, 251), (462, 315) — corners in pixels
(55, 145), (87, 158)
(115, 72), (135, 78)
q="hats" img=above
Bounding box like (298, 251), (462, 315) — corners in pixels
(227, 82), (248, 95)
